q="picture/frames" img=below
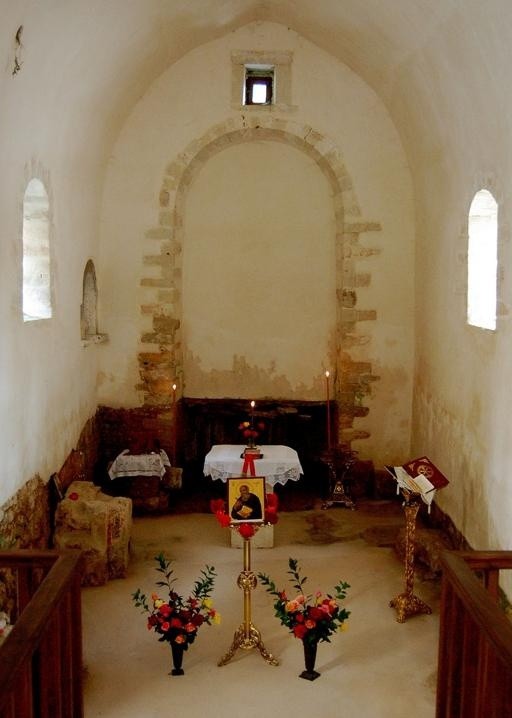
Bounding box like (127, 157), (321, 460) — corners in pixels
(227, 476), (266, 526)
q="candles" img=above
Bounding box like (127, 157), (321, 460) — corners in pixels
(171, 383), (177, 466)
(325, 370), (331, 452)
(250, 400), (256, 428)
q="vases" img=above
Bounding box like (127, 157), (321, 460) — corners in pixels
(298, 639), (321, 681)
(217, 541), (279, 668)
(168, 645), (185, 676)
(247, 438), (256, 448)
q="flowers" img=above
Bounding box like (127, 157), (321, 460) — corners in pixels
(209, 492), (280, 539)
(129, 551), (222, 651)
(255, 555), (353, 644)
(237, 421), (265, 439)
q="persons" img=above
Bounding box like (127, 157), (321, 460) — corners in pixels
(230, 485), (263, 520)
